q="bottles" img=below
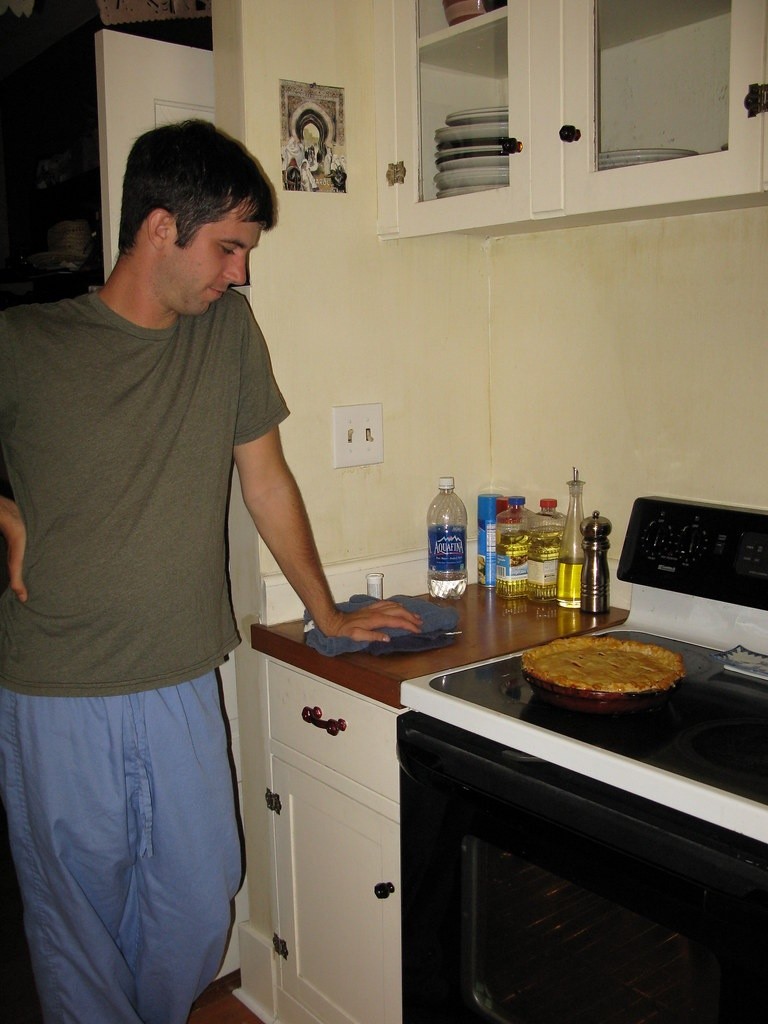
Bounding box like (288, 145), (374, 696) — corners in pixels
(497, 497), (535, 600)
(557, 468), (586, 608)
(477, 494), (504, 587)
(527, 498), (567, 602)
(427, 477), (468, 600)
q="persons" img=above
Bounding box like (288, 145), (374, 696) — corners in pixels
(0, 118), (426, 1023)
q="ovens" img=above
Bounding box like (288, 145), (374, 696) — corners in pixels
(396, 710), (768, 1024)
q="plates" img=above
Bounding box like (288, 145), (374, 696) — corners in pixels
(597, 148), (699, 170)
(434, 106), (509, 199)
(520, 673), (669, 699)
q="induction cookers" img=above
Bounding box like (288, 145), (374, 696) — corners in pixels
(401, 496), (768, 846)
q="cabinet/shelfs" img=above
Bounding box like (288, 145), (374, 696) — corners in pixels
(378, 0), (768, 241)
(260, 655), (403, 1024)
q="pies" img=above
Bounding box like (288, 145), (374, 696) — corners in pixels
(521, 636), (686, 694)
